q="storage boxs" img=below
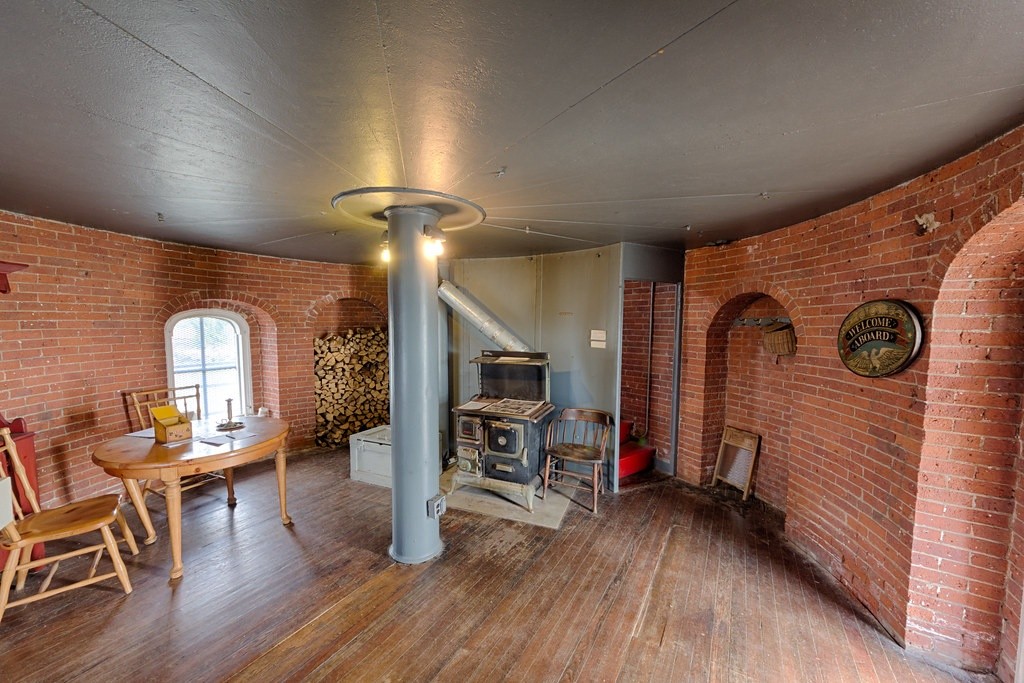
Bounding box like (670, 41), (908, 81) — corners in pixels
(151, 405), (192, 442)
(350, 425), (392, 488)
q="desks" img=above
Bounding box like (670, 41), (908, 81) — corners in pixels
(92, 414), (289, 576)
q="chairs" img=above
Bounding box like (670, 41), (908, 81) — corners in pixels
(544, 408), (613, 513)
(130, 384), (226, 502)
(0, 427), (139, 623)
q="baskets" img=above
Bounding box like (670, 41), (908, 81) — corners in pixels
(761, 322), (797, 355)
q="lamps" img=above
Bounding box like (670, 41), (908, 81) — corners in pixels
(380, 229), (388, 247)
(425, 224), (446, 242)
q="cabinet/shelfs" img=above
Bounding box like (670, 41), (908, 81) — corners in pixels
(0, 416), (47, 573)
